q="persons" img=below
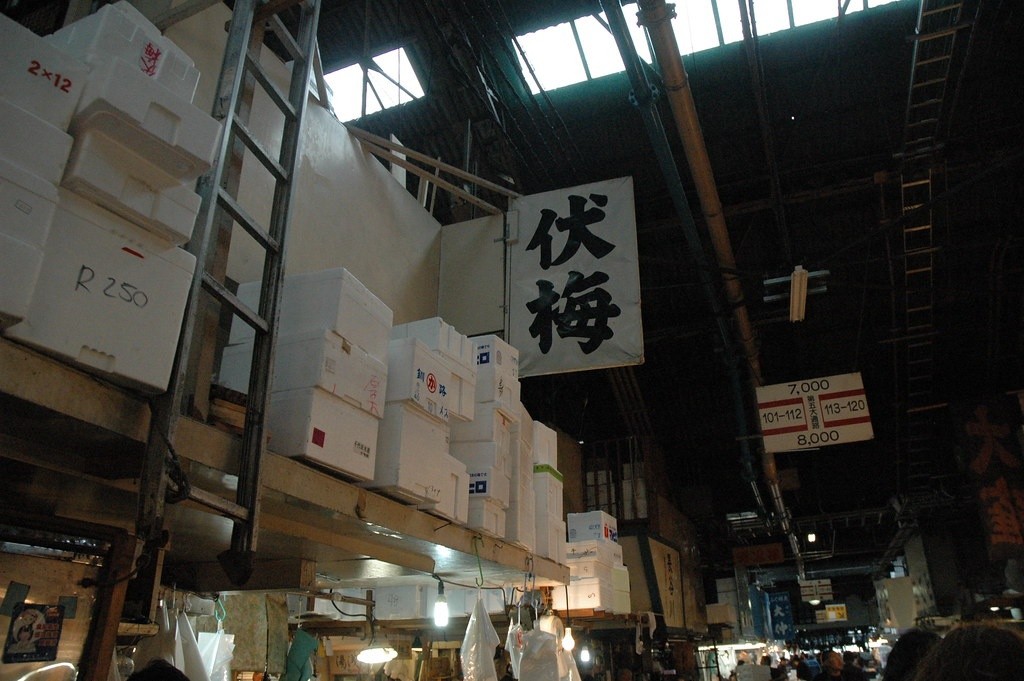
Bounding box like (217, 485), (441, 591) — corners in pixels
(616, 669), (633, 681)
(729, 654), (814, 681)
(126, 656), (190, 681)
(882, 627), (943, 681)
(493, 655), (518, 681)
(908, 619), (1024, 681)
(800, 650), (870, 681)
(653, 657), (662, 674)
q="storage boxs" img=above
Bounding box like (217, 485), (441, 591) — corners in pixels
(0, 0), (632, 618)
(706, 603), (737, 624)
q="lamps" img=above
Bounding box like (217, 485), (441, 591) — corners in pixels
(356, 619), (398, 664)
(411, 631), (423, 651)
